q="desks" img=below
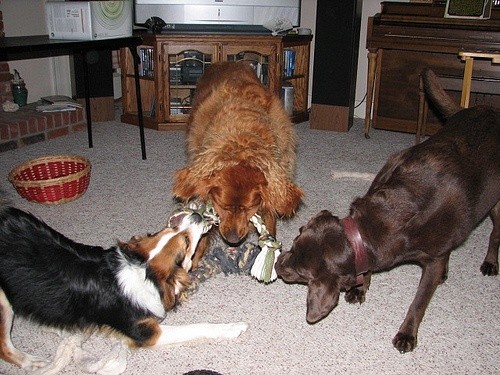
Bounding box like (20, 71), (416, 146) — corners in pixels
(0, 33), (151, 160)
(414, 70), (500, 145)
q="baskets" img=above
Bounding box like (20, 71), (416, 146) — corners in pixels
(8, 154), (93, 206)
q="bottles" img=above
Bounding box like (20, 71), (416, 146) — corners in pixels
(12, 83), (28, 106)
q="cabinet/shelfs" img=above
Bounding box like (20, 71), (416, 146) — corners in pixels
(119, 27), (313, 131)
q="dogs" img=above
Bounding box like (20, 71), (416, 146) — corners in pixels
(0, 199), (249, 375)
(272, 68), (500, 355)
(174, 59), (305, 248)
